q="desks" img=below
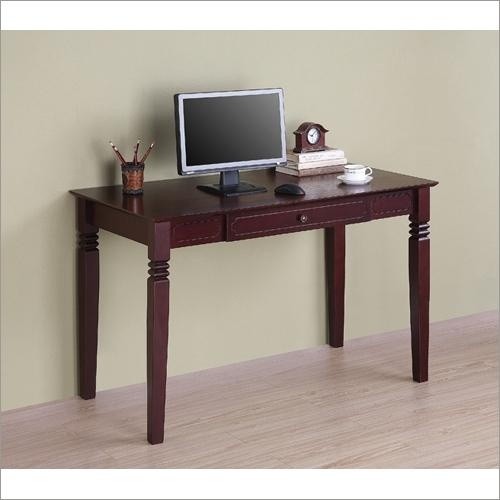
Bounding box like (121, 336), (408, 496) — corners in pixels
(66, 163), (439, 445)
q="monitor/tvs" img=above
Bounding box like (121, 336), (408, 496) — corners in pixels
(173, 87), (288, 197)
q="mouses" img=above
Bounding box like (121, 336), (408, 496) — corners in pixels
(275, 183), (306, 197)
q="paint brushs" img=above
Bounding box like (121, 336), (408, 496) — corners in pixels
(109, 138), (155, 166)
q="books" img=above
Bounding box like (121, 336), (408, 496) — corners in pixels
(286, 146), (345, 164)
(277, 158), (349, 171)
(274, 164), (347, 178)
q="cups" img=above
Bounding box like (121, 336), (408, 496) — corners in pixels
(120, 162), (145, 196)
(342, 164), (374, 181)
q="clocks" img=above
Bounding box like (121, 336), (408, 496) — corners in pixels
(293, 122), (328, 153)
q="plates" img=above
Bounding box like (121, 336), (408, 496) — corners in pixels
(336, 174), (374, 185)
